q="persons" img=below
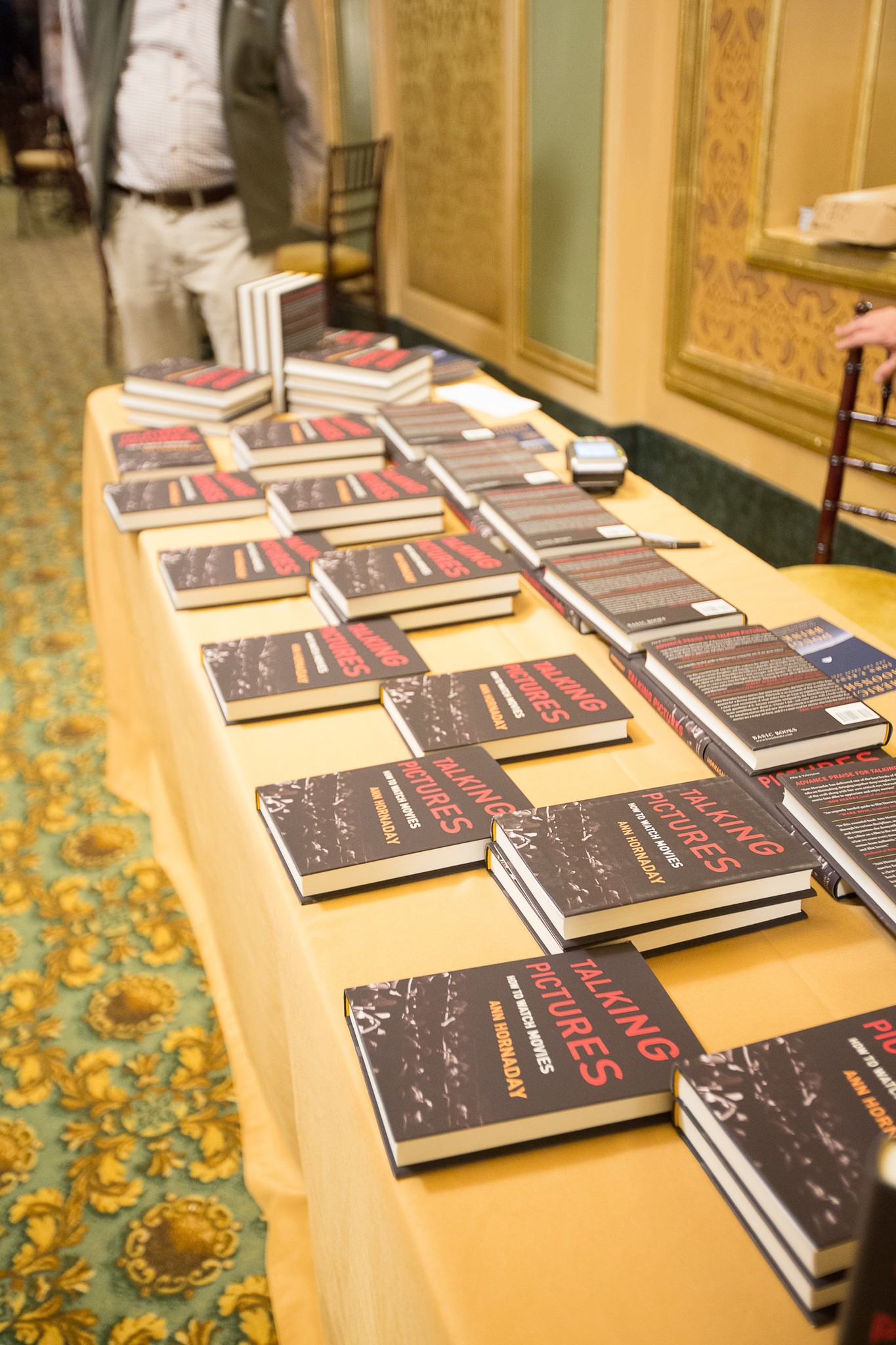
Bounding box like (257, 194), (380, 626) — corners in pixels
(58, 0), (326, 373)
(834, 304), (896, 382)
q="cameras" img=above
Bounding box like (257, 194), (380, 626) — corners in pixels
(564, 433), (632, 493)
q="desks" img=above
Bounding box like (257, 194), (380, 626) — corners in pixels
(751, 224), (896, 295)
(80, 359), (896, 1345)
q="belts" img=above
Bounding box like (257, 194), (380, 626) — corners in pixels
(111, 180), (238, 212)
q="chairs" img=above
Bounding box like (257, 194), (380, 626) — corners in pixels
(782, 303), (896, 657)
(0, 88), (90, 233)
(258, 138), (388, 335)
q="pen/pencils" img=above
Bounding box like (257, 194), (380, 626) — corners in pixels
(644, 540), (701, 549)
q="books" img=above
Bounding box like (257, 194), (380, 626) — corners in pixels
(100, 268), (896, 1345)
